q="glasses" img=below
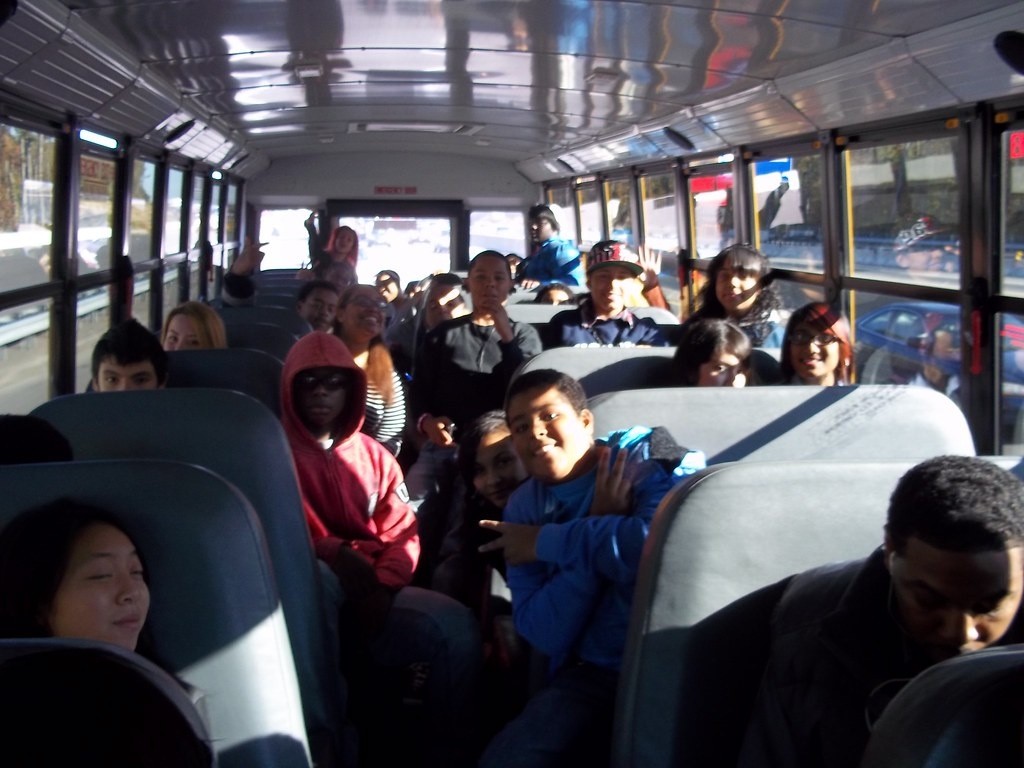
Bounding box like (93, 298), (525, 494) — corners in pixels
(788, 331), (839, 346)
(343, 295), (389, 312)
(297, 375), (346, 388)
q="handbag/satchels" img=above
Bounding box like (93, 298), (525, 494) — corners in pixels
(294, 259), (320, 282)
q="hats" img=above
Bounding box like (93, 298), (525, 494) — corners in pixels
(547, 203), (567, 228)
(585, 240), (643, 277)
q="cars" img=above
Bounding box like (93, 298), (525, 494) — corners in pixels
(855, 297), (1024, 444)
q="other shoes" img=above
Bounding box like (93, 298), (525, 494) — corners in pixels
(402, 663), (430, 705)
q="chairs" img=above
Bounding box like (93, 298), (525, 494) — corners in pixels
(0, 268), (351, 768)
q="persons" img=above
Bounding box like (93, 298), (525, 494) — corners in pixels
(91, 204), (857, 768)
(0, 499), (208, 730)
(0, 414), (74, 464)
(733, 460), (1024, 768)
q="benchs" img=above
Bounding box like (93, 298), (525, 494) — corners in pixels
(411, 271), (1024, 768)
